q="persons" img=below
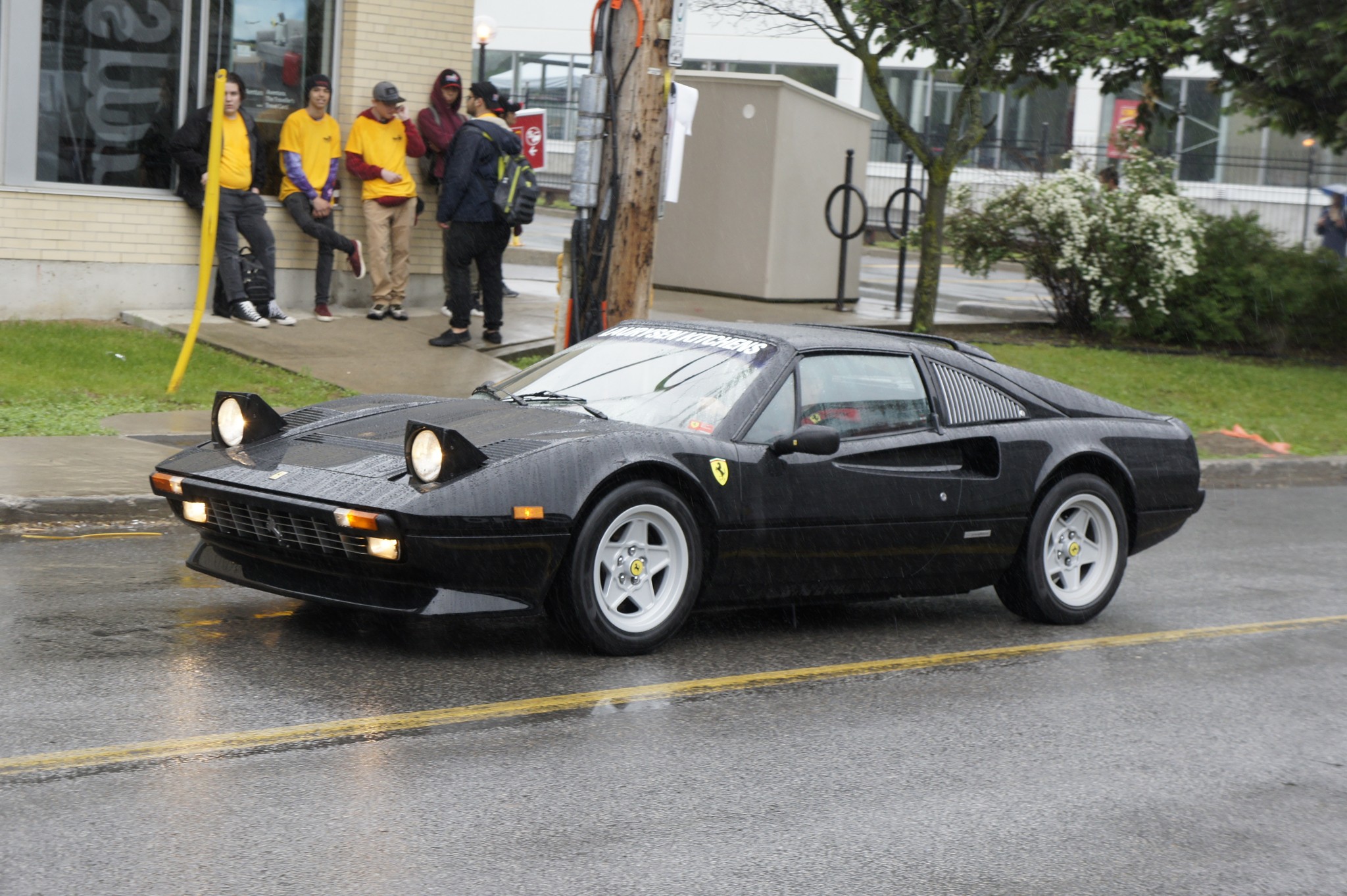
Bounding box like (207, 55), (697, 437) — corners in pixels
(427, 80), (521, 347)
(163, 72), (298, 327)
(1312, 192), (1347, 259)
(277, 73), (368, 321)
(495, 95), (523, 294)
(419, 68), (486, 317)
(344, 80), (424, 321)
(1096, 168), (1135, 320)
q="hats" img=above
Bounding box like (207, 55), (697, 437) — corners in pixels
(505, 99), (523, 113)
(439, 71), (462, 90)
(470, 80), (504, 112)
(304, 74), (333, 100)
(372, 81), (405, 105)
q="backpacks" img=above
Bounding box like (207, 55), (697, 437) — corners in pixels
(475, 141), (539, 228)
(212, 246), (272, 322)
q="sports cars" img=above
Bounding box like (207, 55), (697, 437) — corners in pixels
(147, 318), (1207, 654)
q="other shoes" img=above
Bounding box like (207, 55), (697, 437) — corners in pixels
(502, 281), (519, 298)
(389, 305), (409, 320)
(482, 330), (501, 345)
(429, 328), (471, 347)
(470, 298), (485, 317)
(441, 300), (453, 316)
(366, 303), (389, 320)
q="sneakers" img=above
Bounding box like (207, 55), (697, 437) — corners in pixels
(345, 240), (366, 279)
(254, 300), (297, 326)
(230, 300), (270, 329)
(313, 303), (333, 322)
